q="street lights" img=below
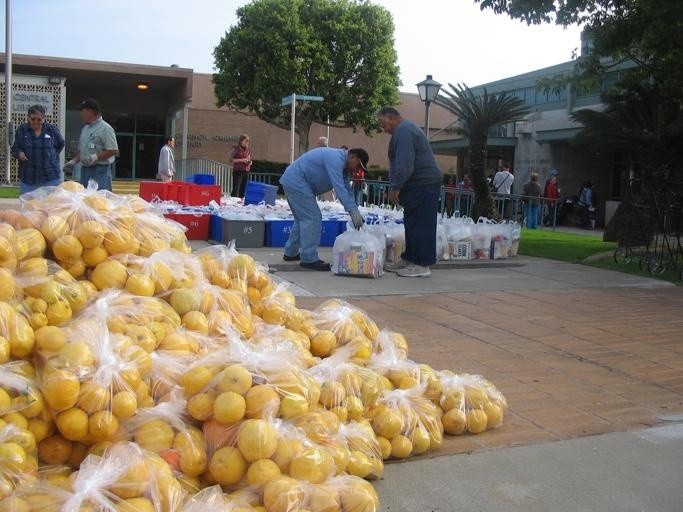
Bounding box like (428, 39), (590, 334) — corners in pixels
(417, 75), (442, 145)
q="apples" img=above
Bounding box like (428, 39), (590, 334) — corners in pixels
(1, 179), (507, 511)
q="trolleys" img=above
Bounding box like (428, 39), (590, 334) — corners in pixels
(614, 177), (682, 275)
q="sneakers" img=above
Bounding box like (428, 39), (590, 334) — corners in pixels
(282, 252), (300, 261)
(300, 259), (331, 271)
(384, 259), (411, 273)
(396, 262), (432, 278)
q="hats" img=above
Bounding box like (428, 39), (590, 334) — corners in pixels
(352, 147), (370, 174)
(74, 98), (101, 111)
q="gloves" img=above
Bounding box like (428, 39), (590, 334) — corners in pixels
(79, 153), (98, 168)
(61, 159), (76, 174)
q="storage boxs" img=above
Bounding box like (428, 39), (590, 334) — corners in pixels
(139, 181), (168, 203)
(267, 218), (339, 246)
(340, 219), (405, 243)
(180, 185), (221, 206)
(222, 216), (265, 248)
(184, 174), (217, 185)
(162, 212), (212, 240)
(244, 181), (279, 205)
(212, 211), (222, 241)
(168, 180), (194, 201)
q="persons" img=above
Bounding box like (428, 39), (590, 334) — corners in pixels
(353, 168), (401, 210)
(279, 147), (369, 270)
(578, 181), (597, 215)
(11, 105), (65, 193)
(63, 97), (118, 192)
(377, 106), (441, 277)
(314, 137), (336, 202)
(230, 134), (252, 198)
(444, 176), (474, 218)
(523, 169), (561, 229)
(493, 163), (515, 213)
(156, 136), (175, 182)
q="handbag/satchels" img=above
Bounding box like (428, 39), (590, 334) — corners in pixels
(490, 185), (499, 197)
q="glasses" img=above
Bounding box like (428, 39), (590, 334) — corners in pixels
(28, 117), (43, 122)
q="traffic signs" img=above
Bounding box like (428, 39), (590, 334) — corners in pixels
(294, 95), (324, 101)
(281, 95), (291, 106)
